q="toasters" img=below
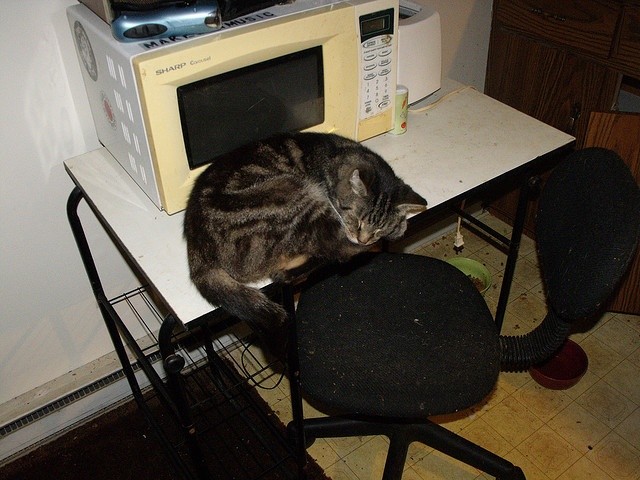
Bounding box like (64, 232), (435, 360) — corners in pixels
(399, 1), (443, 110)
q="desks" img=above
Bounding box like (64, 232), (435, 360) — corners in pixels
(63, 74), (576, 403)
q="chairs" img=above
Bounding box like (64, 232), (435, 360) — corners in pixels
(288, 146), (640, 480)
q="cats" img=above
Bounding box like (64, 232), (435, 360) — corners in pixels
(183, 131), (428, 328)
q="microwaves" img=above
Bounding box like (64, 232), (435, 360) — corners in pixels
(66, 2), (398, 216)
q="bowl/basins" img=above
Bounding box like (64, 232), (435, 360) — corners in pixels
(527, 337), (588, 390)
(443, 256), (492, 297)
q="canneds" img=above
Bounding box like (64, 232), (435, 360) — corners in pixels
(391, 84), (408, 135)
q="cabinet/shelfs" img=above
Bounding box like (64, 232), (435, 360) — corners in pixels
(485, 1), (640, 317)
(66, 186), (307, 479)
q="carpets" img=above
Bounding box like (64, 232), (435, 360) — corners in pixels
(1, 357), (322, 479)
